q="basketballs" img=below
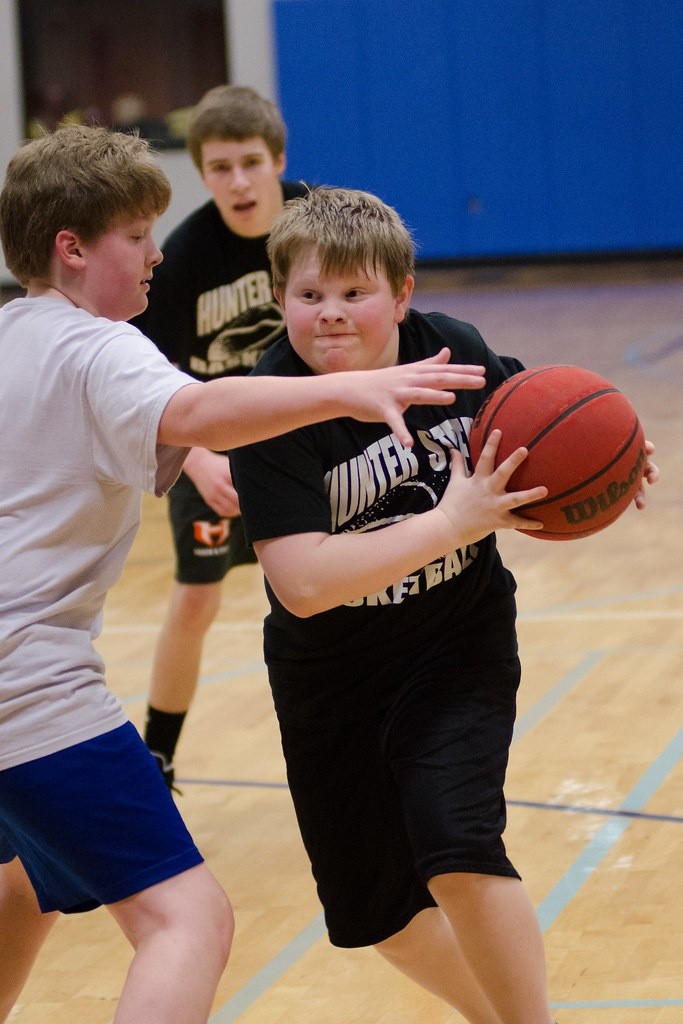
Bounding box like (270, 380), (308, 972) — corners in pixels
(468, 364), (644, 541)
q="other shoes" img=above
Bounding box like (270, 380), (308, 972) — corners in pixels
(149, 752), (183, 798)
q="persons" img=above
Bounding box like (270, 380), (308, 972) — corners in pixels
(136, 83), (314, 794)
(0, 123), (484, 1024)
(225, 180), (658, 1024)
